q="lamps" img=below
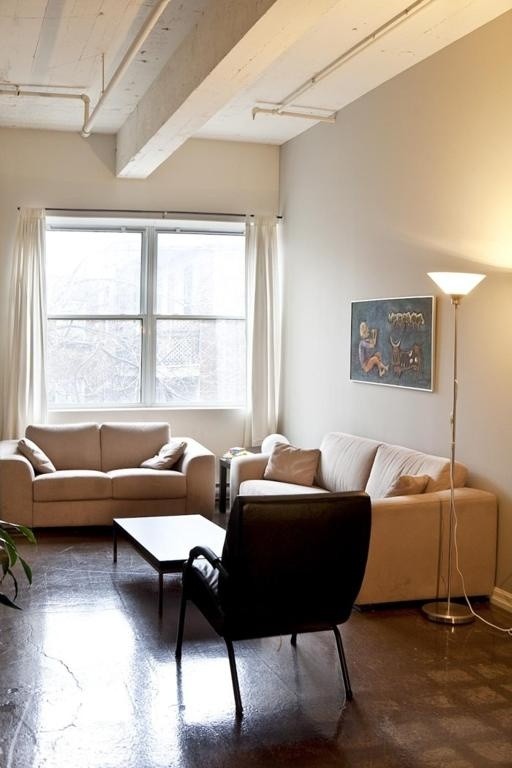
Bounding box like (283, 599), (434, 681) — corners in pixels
(423, 271), (487, 625)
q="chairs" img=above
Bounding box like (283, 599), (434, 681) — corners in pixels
(176, 490), (373, 719)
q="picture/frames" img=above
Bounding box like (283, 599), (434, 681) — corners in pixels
(348, 296), (438, 393)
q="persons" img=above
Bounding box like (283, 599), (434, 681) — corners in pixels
(359, 322), (389, 377)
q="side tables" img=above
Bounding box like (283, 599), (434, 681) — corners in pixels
(219, 446), (261, 513)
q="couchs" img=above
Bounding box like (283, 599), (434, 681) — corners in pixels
(227, 430), (499, 607)
(0, 421), (216, 528)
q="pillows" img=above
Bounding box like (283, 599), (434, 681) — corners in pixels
(381, 474), (430, 498)
(17, 438), (56, 473)
(263, 442), (321, 487)
(140, 439), (188, 470)
